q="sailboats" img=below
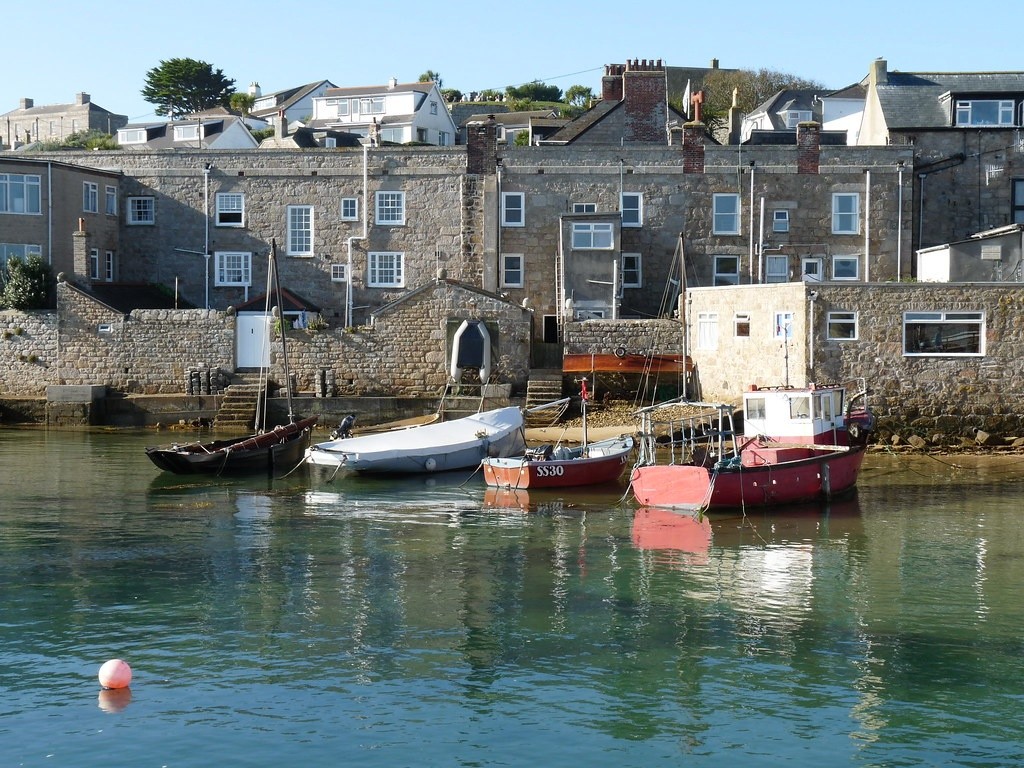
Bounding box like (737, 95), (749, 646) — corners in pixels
(144, 236), (320, 478)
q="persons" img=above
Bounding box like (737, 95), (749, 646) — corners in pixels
(341, 414), (355, 439)
(555, 439), (574, 460)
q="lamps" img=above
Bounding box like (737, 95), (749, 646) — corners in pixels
(750, 160), (755, 166)
(204, 163), (210, 169)
(898, 159), (904, 165)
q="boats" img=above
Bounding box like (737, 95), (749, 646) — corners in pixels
(480, 375), (635, 489)
(628, 229), (870, 510)
(304, 396), (572, 472)
(630, 488), (865, 557)
(480, 481), (622, 509)
